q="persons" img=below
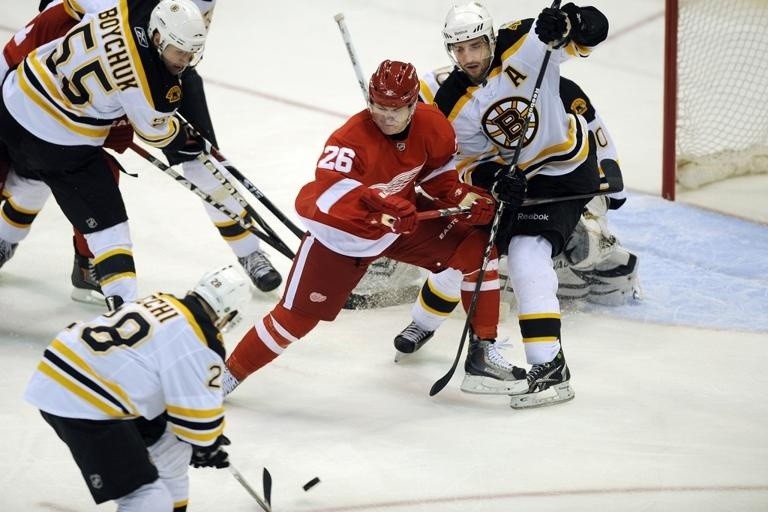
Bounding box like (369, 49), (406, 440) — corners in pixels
(21, 264), (254, 512)
(0, 1), (207, 303)
(223, 60), (529, 399)
(159, 1), (283, 292)
(0, 1), (134, 305)
(391, 1), (609, 409)
(414, 63), (644, 307)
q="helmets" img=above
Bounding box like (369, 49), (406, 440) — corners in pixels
(442, 2), (496, 67)
(367, 60), (421, 109)
(189, 263), (254, 334)
(147, 0), (207, 68)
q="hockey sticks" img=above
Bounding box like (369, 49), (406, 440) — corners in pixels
(178, 111), (401, 279)
(430, 1), (561, 398)
(129, 141), (419, 311)
(224, 463), (276, 511)
(129, 140), (418, 307)
(388, 158), (625, 227)
(191, 146), (296, 259)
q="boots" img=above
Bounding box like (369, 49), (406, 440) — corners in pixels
(236, 251), (282, 301)
(459, 336), (531, 396)
(510, 356), (577, 410)
(392, 320), (435, 365)
(70, 238), (109, 307)
(0, 239), (19, 269)
(599, 245), (646, 308)
